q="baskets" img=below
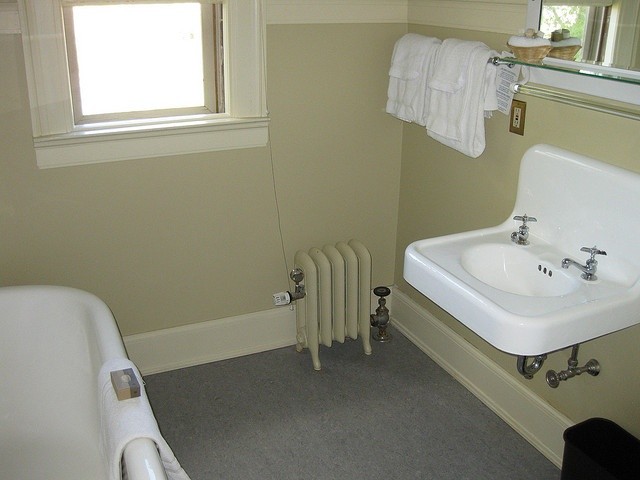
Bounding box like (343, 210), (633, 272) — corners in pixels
(548, 45), (582, 60)
(507, 42), (554, 64)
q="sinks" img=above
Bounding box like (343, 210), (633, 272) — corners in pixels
(406, 225), (637, 357)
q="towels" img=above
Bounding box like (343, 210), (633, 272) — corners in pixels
(93, 356), (195, 480)
(425, 36), (501, 159)
(385, 31), (443, 128)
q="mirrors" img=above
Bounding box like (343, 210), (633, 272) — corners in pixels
(525, 0), (640, 79)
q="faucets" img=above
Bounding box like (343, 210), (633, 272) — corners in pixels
(511, 226), (529, 245)
(561, 257), (598, 280)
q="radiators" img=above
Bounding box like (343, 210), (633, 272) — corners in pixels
(272, 239), (373, 371)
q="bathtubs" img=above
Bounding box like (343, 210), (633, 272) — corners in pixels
(1, 283), (169, 480)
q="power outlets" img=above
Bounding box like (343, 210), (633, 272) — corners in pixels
(509, 99), (527, 136)
